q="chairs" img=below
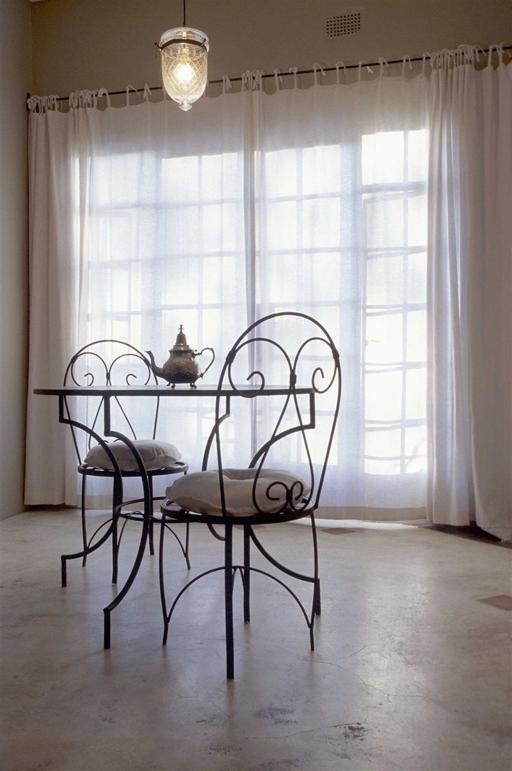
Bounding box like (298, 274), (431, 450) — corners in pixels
(158, 312), (342, 679)
(58, 339), (191, 650)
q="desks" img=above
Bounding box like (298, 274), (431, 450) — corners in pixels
(33, 384), (315, 645)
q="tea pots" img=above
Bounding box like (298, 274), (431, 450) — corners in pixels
(144, 324), (215, 388)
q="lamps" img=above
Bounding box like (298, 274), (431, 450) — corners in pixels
(155, 1), (210, 112)
